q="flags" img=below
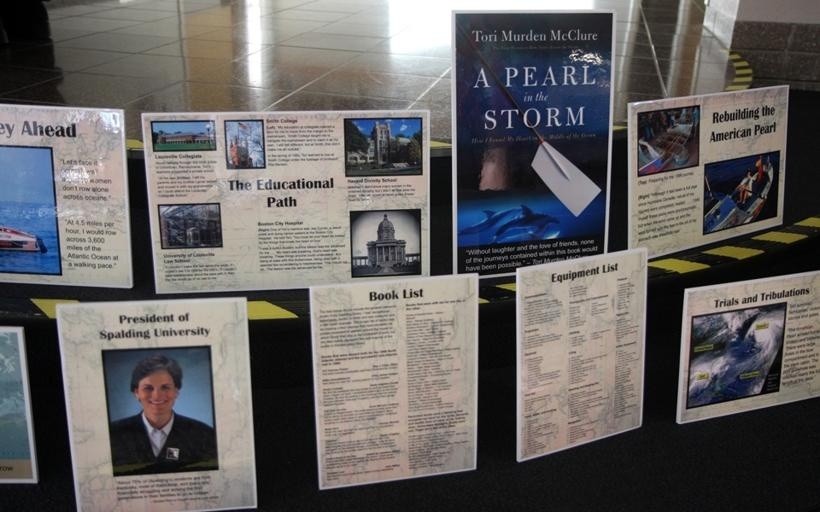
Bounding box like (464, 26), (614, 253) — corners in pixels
(238, 121), (246, 130)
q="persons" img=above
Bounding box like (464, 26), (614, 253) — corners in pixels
(107, 353), (220, 476)
(645, 107), (698, 141)
(735, 169), (755, 206)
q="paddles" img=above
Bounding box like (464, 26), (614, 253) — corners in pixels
(455, 17), (602, 218)
